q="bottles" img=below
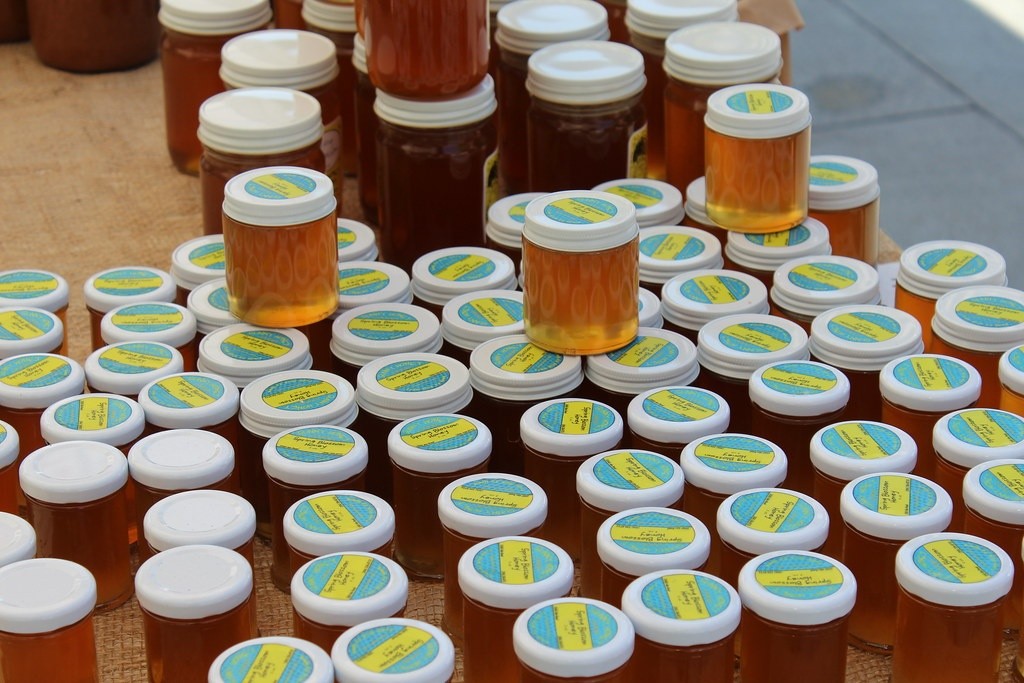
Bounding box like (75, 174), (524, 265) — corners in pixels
(0, 558), (99, 683)
(388, 413), (493, 578)
(307, 216), (474, 506)
(208, 618), (455, 683)
(158, 0), (344, 236)
(0, 421), (37, 567)
(128, 372), (244, 567)
(86, 266), (196, 402)
(222, 166), (340, 328)
(273, 0), (782, 275)
(810, 285), (1024, 683)
(170, 233), (359, 541)
(262, 425), (408, 658)
(0, 0), (158, 75)
(0, 269), (85, 505)
(19, 441), (134, 616)
(412, 193), (830, 660)
(621, 550), (858, 683)
(458, 535), (636, 683)
(521, 190), (640, 356)
(40, 393), (147, 545)
(438, 472), (549, 650)
(134, 490), (259, 683)
(594, 155), (1007, 498)
(703, 83), (812, 234)
